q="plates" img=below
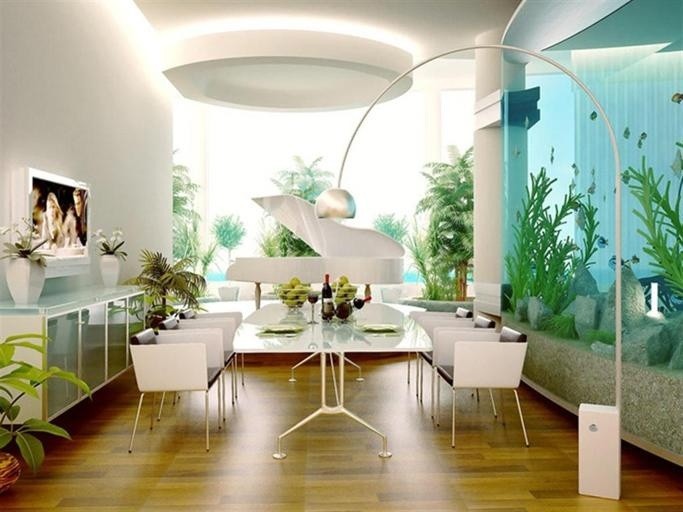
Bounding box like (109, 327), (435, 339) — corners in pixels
(263, 324), (302, 334)
(363, 323), (398, 333)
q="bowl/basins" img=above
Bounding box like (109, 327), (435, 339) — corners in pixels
(329, 285), (358, 304)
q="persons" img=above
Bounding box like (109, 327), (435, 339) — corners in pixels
(45, 193), (64, 249)
(30, 187), (44, 242)
(61, 204), (78, 237)
(71, 188), (86, 245)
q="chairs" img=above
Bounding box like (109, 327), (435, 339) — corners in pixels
(407, 306), (531, 448)
(126, 306), (245, 453)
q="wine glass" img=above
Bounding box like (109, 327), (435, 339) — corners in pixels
(272, 284), (312, 316)
(307, 293), (319, 325)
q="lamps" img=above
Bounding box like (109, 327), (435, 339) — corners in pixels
(314, 43), (624, 502)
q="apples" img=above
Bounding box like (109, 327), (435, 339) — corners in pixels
(332, 276), (356, 304)
(278, 277), (309, 305)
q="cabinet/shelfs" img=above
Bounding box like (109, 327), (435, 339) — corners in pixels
(1, 287), (146, 426)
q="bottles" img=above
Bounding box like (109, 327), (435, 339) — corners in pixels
(349, 296), (371, 311)
(321, 274), (333, 321)
(363, 283), (371, 303)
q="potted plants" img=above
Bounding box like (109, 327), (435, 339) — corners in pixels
(1, 332), (94, 495)
(0, 217), (57, 309)
(97, 231), (129, 288)
